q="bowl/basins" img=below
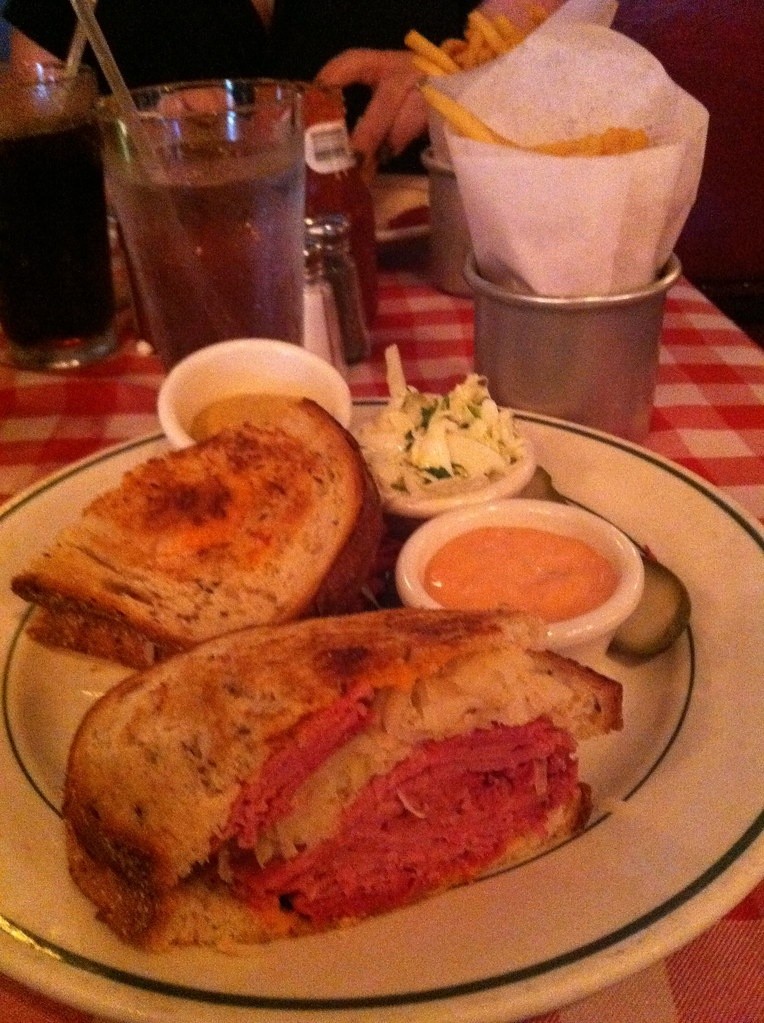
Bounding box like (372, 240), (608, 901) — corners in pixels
(360, 414), (536, 519)
(158, 339), (350, 449)
(396, 498), (644, 667)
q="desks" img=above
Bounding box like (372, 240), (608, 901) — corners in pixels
(0, 266), (764, 1022)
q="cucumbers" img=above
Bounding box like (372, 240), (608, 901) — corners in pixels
(518, 468), (692, 658)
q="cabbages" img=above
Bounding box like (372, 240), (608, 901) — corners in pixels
(356, 345), (531, 500)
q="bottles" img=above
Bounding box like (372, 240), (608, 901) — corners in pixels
(304, 82), (374, 333)
(302, 239), (346, 373)
(304, 216), (372, 365)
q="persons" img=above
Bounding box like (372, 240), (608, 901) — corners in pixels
(0, 0), (764, 346)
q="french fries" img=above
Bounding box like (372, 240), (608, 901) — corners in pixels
(404, 4), (548, 74)
(421, 83), (651, 157)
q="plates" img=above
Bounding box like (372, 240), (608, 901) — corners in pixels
(0, 396), (763, 1023)
(375, 174), (432, 239)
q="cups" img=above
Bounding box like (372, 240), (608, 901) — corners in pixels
(462, 252), (683, 443)
(421, 148), (476, 295)
(0, 63), (116, 371)
(92, 76), (304, 375)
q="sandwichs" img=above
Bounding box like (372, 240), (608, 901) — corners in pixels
(10, 393), (386, 666)
(59, 603), (623, 951)
